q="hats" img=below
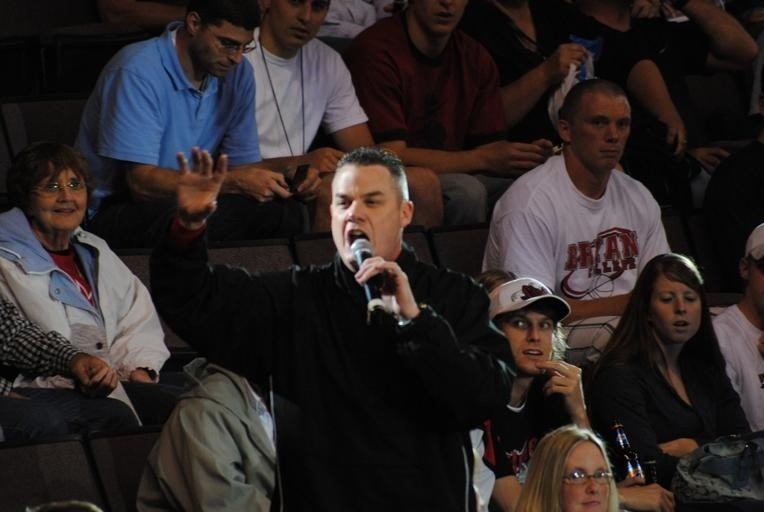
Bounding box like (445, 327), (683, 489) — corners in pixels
(745, 223), (764, 260)
(488, 277), (572, 323)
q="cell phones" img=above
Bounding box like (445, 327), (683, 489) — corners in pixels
(287, 162), (309, 188)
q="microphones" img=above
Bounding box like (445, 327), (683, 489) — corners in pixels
(351, 238), (382, 310)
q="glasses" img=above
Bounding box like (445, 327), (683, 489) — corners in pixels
(202, 20), (257, 53)
(563, 470), (613, 485)
(34, 181), (86, 190)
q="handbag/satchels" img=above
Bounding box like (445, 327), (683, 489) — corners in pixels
(672, 430), (763, 511)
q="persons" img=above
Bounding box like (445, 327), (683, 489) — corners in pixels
(1, 1), (764, 512)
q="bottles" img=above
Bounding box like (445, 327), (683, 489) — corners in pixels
(612, 418), (643, 485)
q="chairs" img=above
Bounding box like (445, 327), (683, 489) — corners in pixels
(0, 29), (143, 194)
(1, 203), (745, 512)
